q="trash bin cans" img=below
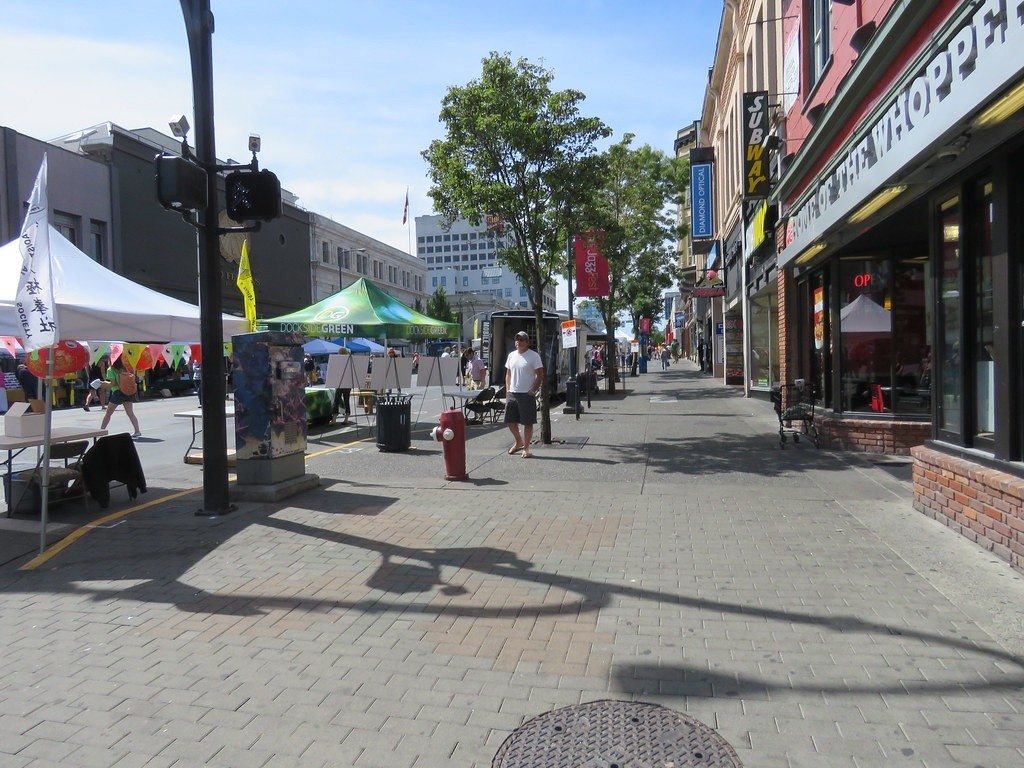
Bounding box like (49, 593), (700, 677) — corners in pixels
(373, 392), (414, 454)
(638, 357), (648, 373)
(626, 354), (631, 365)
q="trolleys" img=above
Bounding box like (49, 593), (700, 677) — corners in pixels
(771, 378), (822, 452)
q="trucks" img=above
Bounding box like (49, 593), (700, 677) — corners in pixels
(487, 307), (579, 399)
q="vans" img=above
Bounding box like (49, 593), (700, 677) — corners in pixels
(426, 339), (469, 358)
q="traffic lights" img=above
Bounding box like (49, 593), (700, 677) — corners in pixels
(154, 155), (209, 212)
(224, 169), (282, 223)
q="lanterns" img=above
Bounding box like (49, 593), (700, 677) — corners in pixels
(25, 340), (90, 406)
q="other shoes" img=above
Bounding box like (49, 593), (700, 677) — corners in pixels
(342, 421), (349, 425)
(131, 432), (141, 437)
(463, 384), (465, 386)
(83, 405), (90, 411)
(329, 422), (337, 426)
(471, 420), (483, 425)
(102, 406), (107, 409)
(456, 384), (459, 386)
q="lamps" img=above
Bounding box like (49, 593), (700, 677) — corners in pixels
(761, 135), (805, 149)
(850, 21), (876, 53)
(970, 78), (1024, 130)
(844, 185), (907, 224)
(806, 103), (825, 124)
(794, 241), (827, 265)
(780, 152), (795, 169)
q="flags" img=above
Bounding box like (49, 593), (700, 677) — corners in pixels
(403, 194), (408, 224)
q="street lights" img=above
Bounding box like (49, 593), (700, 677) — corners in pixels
(459, 290), (478, 342)
(337, 246), (368, 290)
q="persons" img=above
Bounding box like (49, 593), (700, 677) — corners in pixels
(304, 352), (313, 387)
(465, 347), (485, 425)
(461, 346), (469, 386)
(450, 345), (458, 357)
(413, 353), (420, 373)
(83, 360), (106, 411)
(0, 369), (8, 412)
(670, 342), (679, 364)
(442, 347), (450, 357)
(101, 356), (141, 435)
(697, 339), (704, 371)
(328, 348), (350, 424)
(504, 331), (543, 457)
(647, 347), (659, 360)
(660, 345), (669, 370)
(16, 364), (34, 399)
(585, 346), (604, 371)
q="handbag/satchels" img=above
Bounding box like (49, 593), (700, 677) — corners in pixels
(667, 362), (670, 366)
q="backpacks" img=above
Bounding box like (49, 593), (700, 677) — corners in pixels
(112, 367), (137, 395)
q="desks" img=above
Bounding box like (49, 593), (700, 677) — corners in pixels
(174, 405), (235, 464)
(0, 426), (108, 519)
(443, 390), (483, 426)
(305, 388), (345, 426)
(350, 392), (375, 413)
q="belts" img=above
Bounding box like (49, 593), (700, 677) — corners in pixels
(473, 380), (480, 382)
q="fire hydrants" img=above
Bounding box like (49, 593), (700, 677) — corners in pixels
(429, 406), (470, 481)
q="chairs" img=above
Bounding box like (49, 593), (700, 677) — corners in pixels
(9, 433), (132, 518)
(463, 385), (507, 424)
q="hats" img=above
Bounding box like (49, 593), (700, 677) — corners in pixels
(514, 331), (529, 342)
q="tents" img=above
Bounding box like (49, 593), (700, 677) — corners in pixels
(840, 293), (891, 332)
(352, 338), (384, 353)
(0, 222), (249, 554)
(255, 277), (462, 414)
(302, 339), (351, 355)
(330, 338), (370, 372)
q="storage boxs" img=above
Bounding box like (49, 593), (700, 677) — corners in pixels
(4, 399), (45, 438)
(0, 467), (68, 514)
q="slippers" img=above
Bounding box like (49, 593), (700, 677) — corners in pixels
(522, 452), (532, 458)
(508, 446), (524, 454)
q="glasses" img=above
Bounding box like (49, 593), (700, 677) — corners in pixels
(515, 338), (526, 342)
